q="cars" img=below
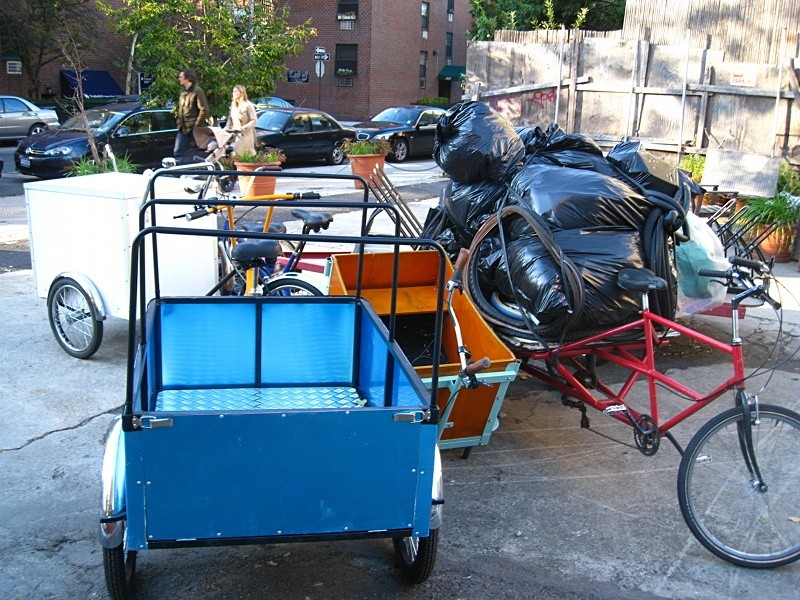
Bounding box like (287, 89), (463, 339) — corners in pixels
(14, 103), (199, 180)
(344, 105), (451, 162)
(0, 94), (63, 147)
(215, 96), (293, 130)
(249, 107), (358, 167)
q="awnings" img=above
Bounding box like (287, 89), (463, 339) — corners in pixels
(438, 64), (466, 81)
(59, 69), (126, 98)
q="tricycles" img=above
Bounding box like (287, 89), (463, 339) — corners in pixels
(501, 268), (798, 567)
(22, 169), (289, 355)
(170, 189), (522, 452)
(97, 161), (445, 600)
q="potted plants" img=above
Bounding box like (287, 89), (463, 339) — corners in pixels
(679, 149), (800, 265)
(228, 145), (287, 197)
(340, 138), (394, 189)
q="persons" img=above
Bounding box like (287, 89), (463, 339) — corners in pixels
(221, 85), (259, 157)
(173, 68), (210, 183)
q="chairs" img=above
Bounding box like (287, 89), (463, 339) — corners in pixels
(294, 119), (305, 133)
